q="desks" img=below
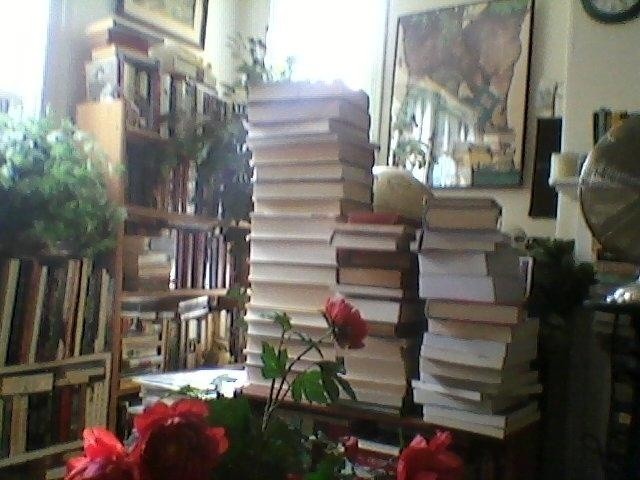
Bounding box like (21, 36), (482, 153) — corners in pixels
(132, 368), (544, 480)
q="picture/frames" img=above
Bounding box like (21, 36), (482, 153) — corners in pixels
(386, 0), (535, 188)
(115, 0), (208, 50)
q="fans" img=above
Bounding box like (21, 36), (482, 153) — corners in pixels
(578, 115), (640, 299)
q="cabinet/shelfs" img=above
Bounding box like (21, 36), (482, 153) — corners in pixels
(0, 16), (244, 479)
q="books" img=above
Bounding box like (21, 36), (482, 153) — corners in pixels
(83, 12), (253, 444)
(412, 194), (545, 442)
(259, 403), (410, 480)
(0, 253), (117, 480)
(330, 210), (418, 414)
(242, 80), (378, 396)
